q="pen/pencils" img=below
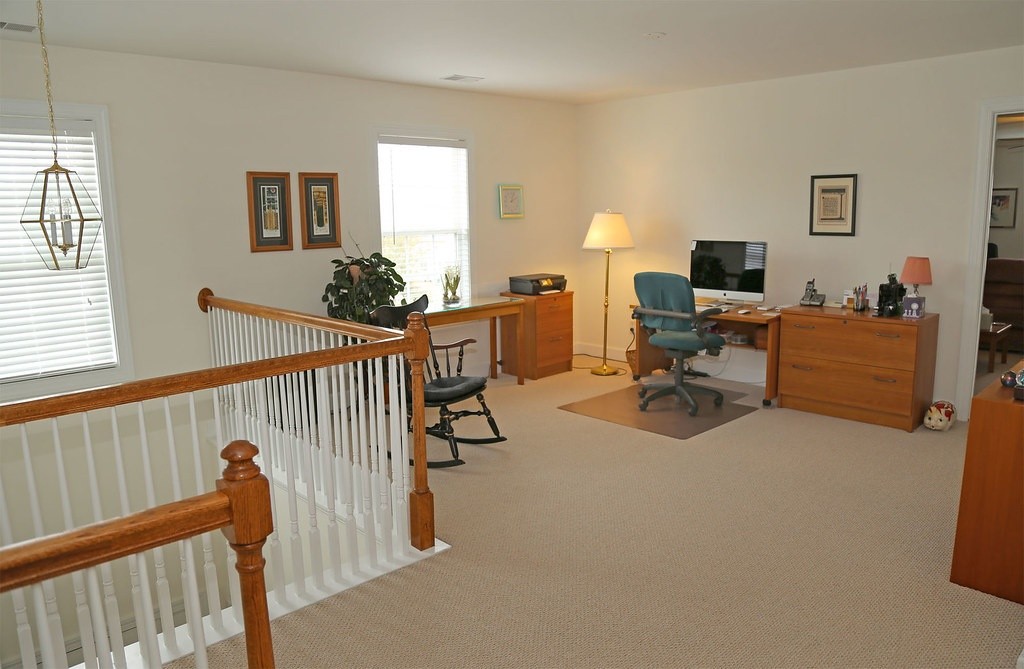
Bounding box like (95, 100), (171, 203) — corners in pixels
(853, 283), (867, 310)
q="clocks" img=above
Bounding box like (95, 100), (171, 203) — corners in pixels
(498, 185), (524, 219)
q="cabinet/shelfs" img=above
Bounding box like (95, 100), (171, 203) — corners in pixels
(949, 359), (1024, 605)
(980, 258), (1024, 353)
(777, 304), (939, 433)
(500, 290), (574, 380)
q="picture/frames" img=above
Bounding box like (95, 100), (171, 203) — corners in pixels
(809, 174), (857, 236)
(990, 187), (1018, 228)
(298, 171), (342, 250)
(246, 171), (294, 253)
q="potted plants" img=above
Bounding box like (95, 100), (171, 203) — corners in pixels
(441, 263), (464, 307)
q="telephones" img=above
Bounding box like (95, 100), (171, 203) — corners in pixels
(800, 278), (826, 305)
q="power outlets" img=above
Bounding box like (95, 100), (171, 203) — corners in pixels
(629, 326), (636, 336)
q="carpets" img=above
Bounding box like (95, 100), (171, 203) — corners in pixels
(556, 384), (759, 440)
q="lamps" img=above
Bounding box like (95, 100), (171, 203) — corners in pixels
(20, 0), (104, 271)
(899, 256), (932, 318)
(580, 208), (635, 376)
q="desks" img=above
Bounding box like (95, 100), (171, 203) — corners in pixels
(384, 295), (526, 404)
(629, 296), (780, 406)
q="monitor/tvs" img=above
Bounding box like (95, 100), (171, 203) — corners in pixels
(690, 240), (767, 303)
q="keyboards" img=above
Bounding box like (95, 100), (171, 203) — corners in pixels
(696, 305), (729, 313)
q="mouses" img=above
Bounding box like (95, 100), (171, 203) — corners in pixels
(738, 310), (751, 314)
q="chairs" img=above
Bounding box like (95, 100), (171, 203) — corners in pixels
(631, 272), (725, 417)
(364, 293), (508, 467)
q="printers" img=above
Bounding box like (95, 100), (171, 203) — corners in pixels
(509, 274), (566, 295)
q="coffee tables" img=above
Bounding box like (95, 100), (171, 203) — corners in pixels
(979, 322), (1013, 372)
